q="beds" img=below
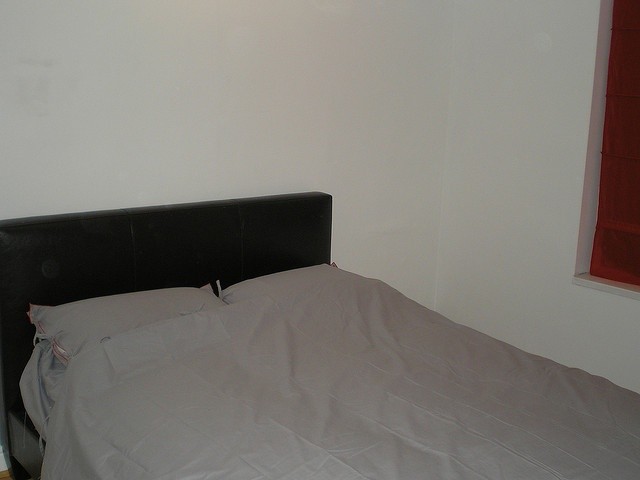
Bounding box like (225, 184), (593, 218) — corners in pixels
(0, 188), (640, 480)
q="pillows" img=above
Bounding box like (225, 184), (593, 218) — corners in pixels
(213, 262), (374, 307)
(21, 283), (239, 368)
